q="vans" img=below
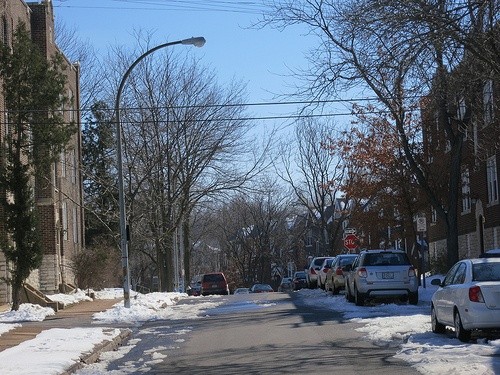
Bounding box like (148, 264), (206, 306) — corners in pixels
(186, 271), (229, 295)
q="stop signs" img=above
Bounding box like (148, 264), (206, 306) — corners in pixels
(344, 235), (360, 250)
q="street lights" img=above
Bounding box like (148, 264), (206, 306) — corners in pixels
(116, 36), (207, 309)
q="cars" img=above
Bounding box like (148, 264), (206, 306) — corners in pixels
(278, 248), (419, 306)
(234, 283), (274, 294)
(430, 257), (500, 342)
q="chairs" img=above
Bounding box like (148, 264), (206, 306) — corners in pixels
(390, 257), (398, 264)
